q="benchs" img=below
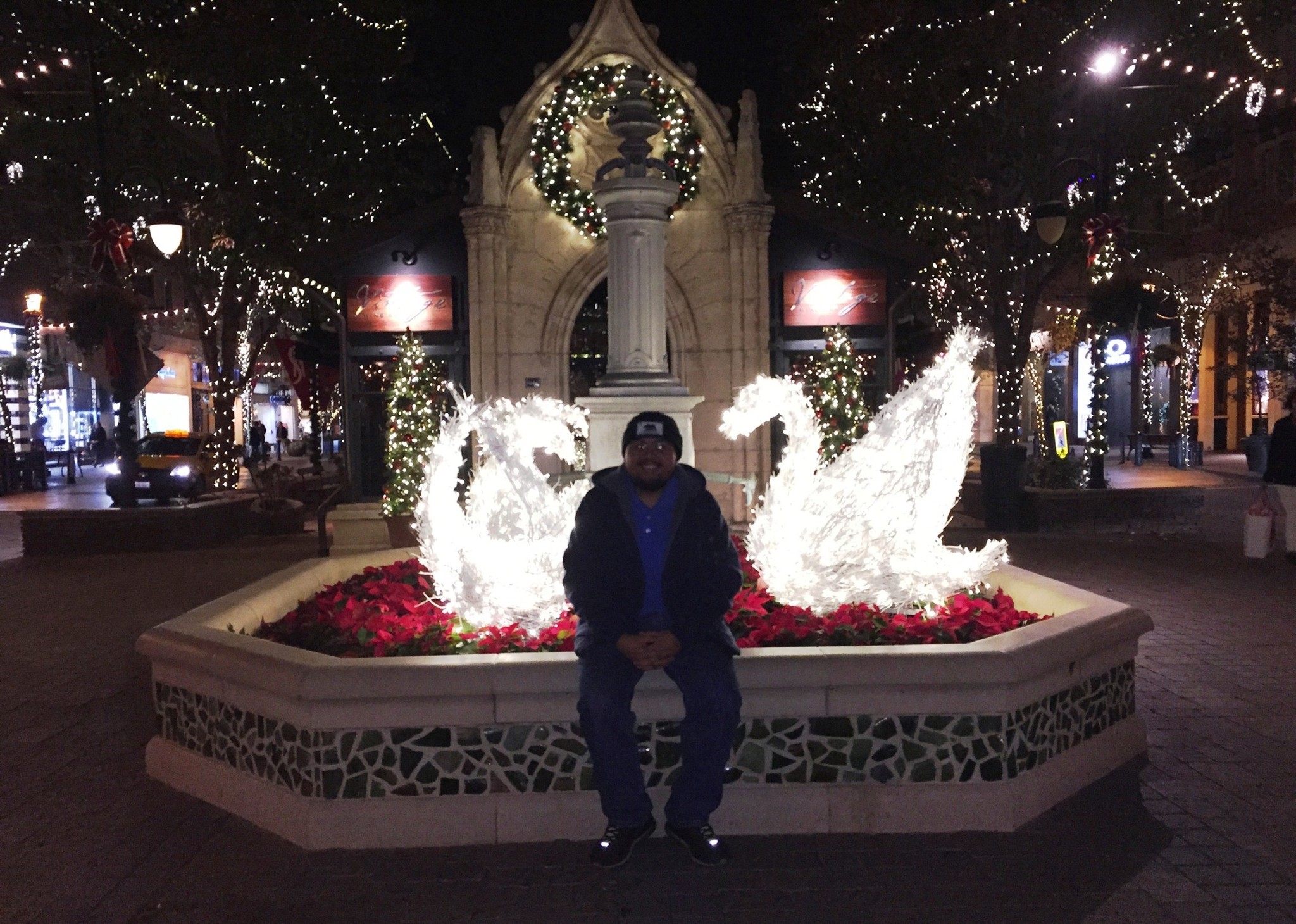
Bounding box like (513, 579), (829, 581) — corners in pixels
(1126, 433), (1168, 461)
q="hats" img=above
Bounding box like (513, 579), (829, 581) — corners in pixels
(621, 411), (683, 460)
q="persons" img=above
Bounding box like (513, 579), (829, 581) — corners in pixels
(1046, 395), (1060, 424)
(88, 422), (107, 469)
(0, 437), (48, 483)
(1259, 389), (1296, 562)
(28, 416), (51, 475)
(275, 421), (288, 456)
(248, 421), (261, 457)
(563, 410), (744, 870)
(256, 420), (267, 456)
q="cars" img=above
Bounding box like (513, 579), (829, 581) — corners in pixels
(106, 429), (240, 504)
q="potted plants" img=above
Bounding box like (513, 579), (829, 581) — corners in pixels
(377, 327), (443, 549)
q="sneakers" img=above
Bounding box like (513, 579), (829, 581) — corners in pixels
(665, 820), (727, 865)
(590, 814), (656, 868)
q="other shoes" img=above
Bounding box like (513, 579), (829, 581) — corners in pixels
(92, 461), (97, 467)
(1285, 550), (1295, 563)
(100, 462), (104, 467)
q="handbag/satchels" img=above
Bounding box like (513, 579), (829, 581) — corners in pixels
(1244, 486), (1277, 558)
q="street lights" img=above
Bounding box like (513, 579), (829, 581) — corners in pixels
(1031, 34), (1141, 489)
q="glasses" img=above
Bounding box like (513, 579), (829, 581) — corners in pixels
(626, 443), (675, 453)
